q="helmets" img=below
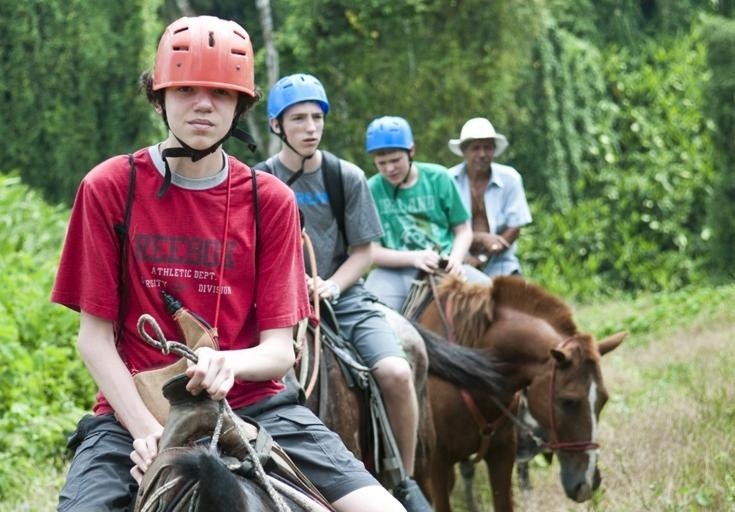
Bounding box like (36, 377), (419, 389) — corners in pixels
(267, 73), (329, 132)
(151, 16), (256, 99)
(365, 116), (414, 153)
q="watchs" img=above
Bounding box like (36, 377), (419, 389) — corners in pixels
(477, 253), (489, 264)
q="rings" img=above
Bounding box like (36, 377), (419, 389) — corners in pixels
(491, 244), (498, 250)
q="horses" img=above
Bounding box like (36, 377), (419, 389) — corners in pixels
(136, 427), (339, 512)
(414, 275), (629, 511)
(300, 298), (510, 512)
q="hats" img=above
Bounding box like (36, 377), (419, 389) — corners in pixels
(447, 117), (509, 158)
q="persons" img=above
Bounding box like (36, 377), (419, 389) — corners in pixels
(53, 13), (409, 512)
(361, 115), (493, 316)
(251, 73), (436, 511)
(445, 117), (533, 280)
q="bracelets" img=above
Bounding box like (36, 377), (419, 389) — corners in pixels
(325, 279), (340, 305)
